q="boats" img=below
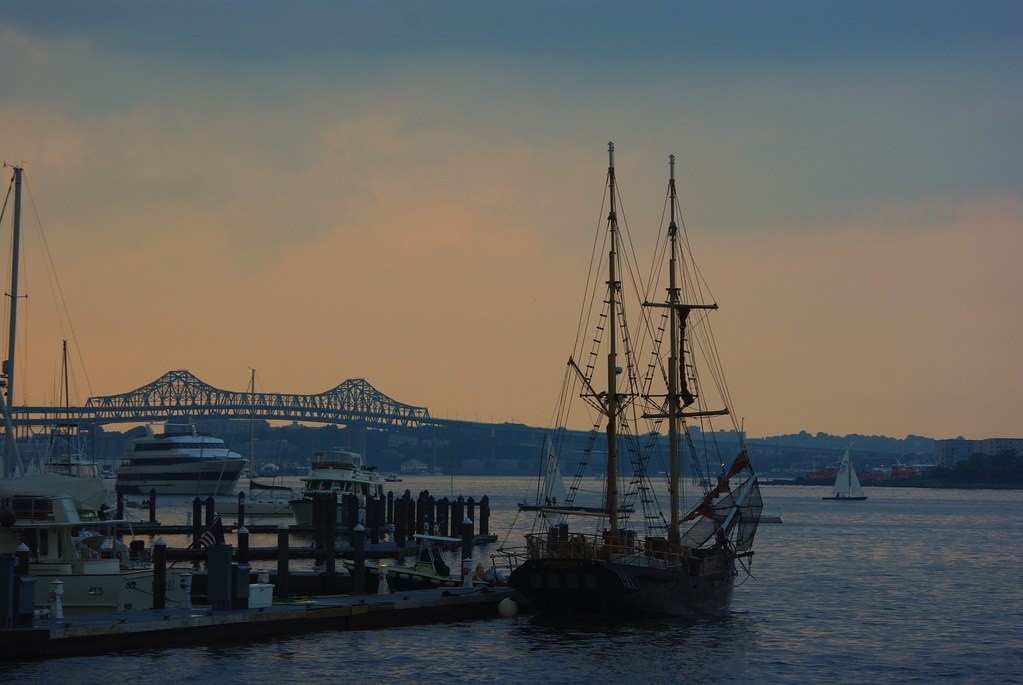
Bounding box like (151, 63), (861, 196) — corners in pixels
(288, 446), (386, 526)
(115, 425), (249, 495)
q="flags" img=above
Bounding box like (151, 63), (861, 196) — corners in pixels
(196, 516), (225, 547)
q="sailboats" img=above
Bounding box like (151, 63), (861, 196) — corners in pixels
(0, 162), (193, 618)
(516, 435), (635, 514)
(822, 445), (868, 500)
(489, 142), (765, 620)
